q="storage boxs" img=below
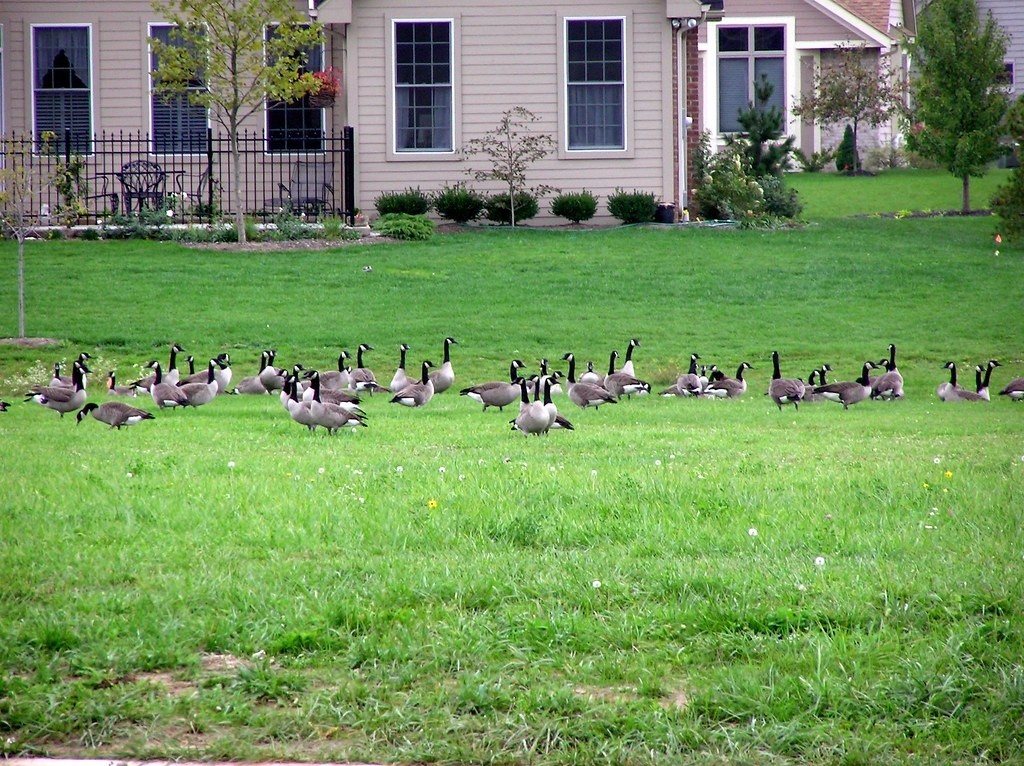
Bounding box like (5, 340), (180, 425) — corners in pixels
(655, 202), (676, 224)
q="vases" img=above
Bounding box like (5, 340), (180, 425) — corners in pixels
(309, 90), (336, 109)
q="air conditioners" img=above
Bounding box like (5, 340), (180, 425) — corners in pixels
(308, 0), (353, 25)
(666, 1), (702, 19)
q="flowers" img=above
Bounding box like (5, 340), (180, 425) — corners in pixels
(356, 211), (364, 221)
(300, 66), (343, 98)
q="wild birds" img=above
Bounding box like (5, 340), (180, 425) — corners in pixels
(0, 336), (1023, 437)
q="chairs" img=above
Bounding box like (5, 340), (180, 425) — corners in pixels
(273, 160), (334, 223)
(75, 175), (119, 216)
(116, 160), (166, 216)
(168, 164), (209, 218)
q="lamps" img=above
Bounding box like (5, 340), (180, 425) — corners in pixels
(671, 19), (697, 28)
